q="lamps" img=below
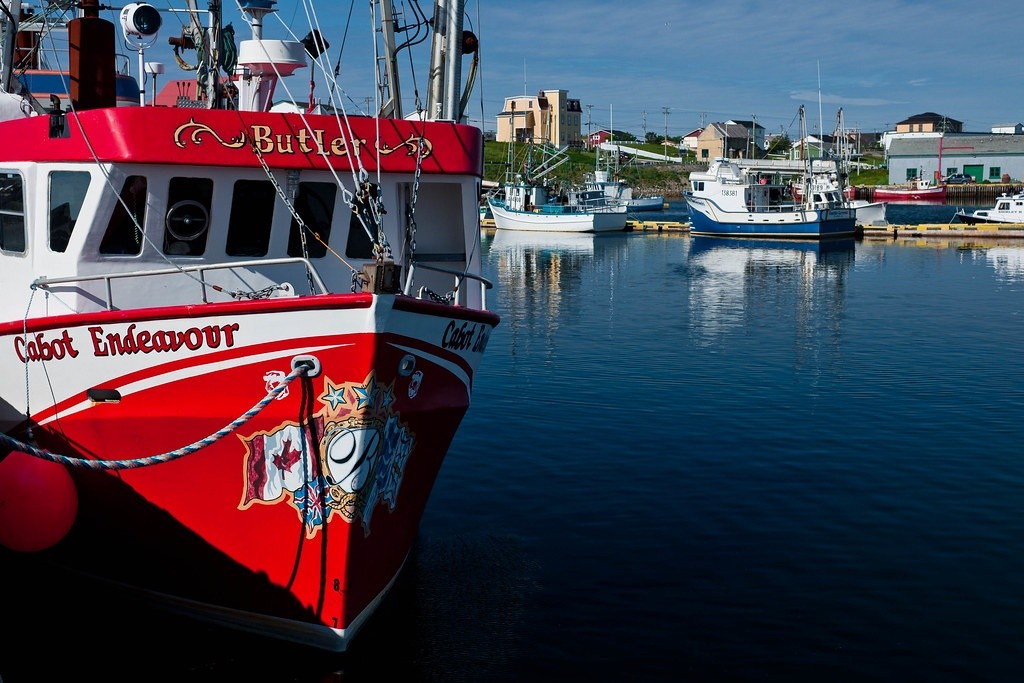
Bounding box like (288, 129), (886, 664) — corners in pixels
(119, 1), (163, 50)
(283, 168), (302, 198)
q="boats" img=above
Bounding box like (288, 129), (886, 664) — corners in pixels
(955, 189), (1024, 226)
(869, 137), (974, 200)
(487, 57), (628, 233)
(828, 107), (888, 225)
(682, 59), (864, 238)
(478, 202), (493, 220)
(592, 104), (663, 213)
(0, 0), (499, 660)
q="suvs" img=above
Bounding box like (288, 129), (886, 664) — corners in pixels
(940, 173), (971, 184)
